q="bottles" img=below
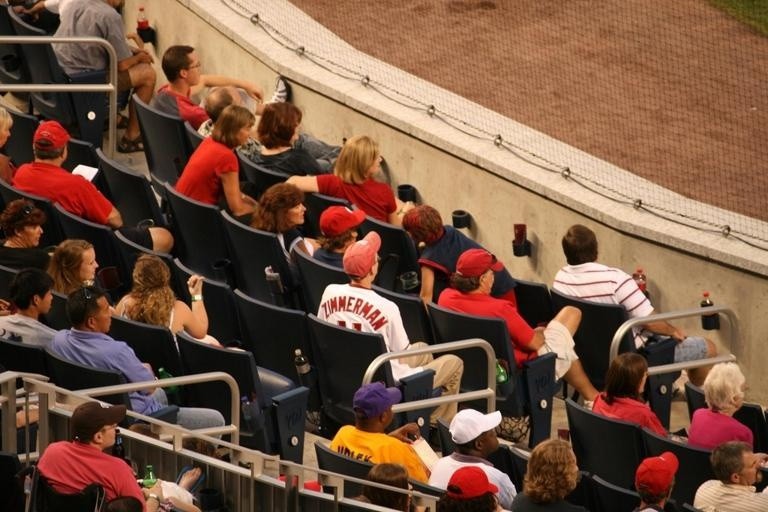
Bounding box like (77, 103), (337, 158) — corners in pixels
(143, 464), (157, 488)
(240, 395), (257, 419)
(158, 367), (177, 393)
(112, 428), (125, 458)
(633, 268), (647, 294)
(136, 8), (148, 29)
(294, 348), (310, 374)
(494, 358), (508, 384)
(700, 291), (716, 317)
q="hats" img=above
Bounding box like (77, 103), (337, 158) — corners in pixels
(446, 466), (499, 500)
(71, 400), (127, 431)
(343, 231), (382, 278)
(350, 382), (402, 418)
(454, 249), (508, 273)
(448, 409), (502, 445)
(319, 205), (366, 237)
(32, 120), (70, 152)
(635, 452), (680, 498)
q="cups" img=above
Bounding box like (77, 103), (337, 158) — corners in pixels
(399, 271), (419, 289)
(513, 223), (526, 244)
(98, 264), (124, 293)
(45, 245), (57, 256)
(136, 217), (154, 233)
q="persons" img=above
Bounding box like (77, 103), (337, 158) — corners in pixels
(1, 1), (767, 511)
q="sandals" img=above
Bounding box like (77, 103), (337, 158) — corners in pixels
(117, 135), (145, 154)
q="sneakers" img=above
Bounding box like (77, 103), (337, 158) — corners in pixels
(671, 383), (687, 401)
(584, 400), (595, 413)
(272, 81), (292, 105)
